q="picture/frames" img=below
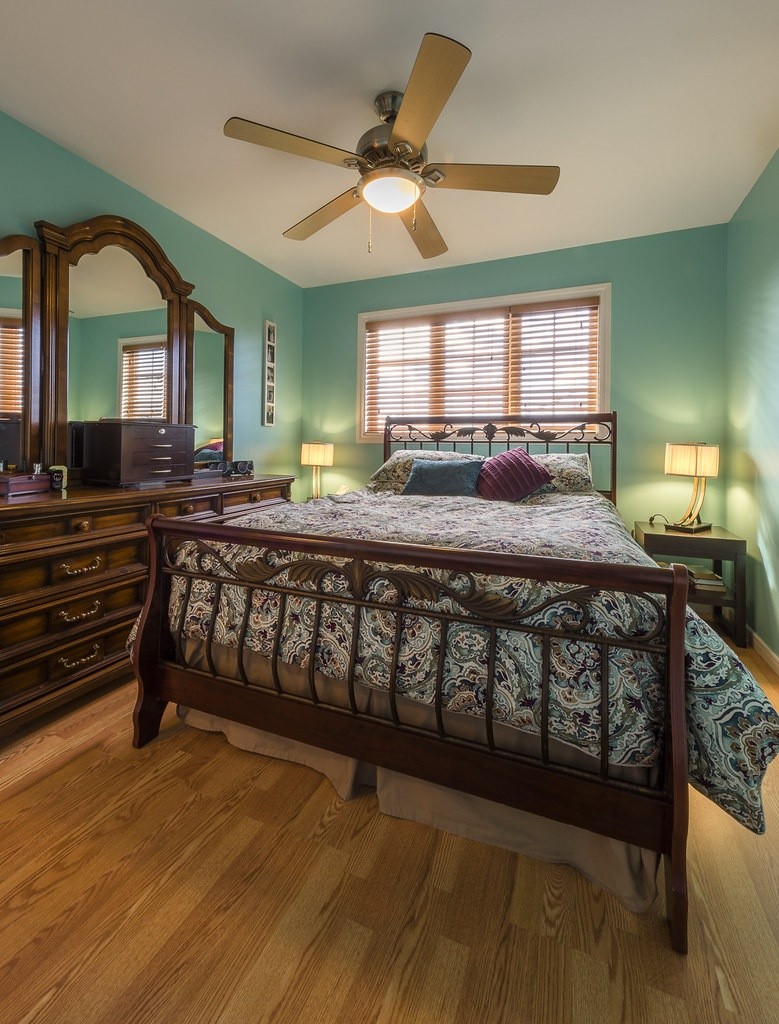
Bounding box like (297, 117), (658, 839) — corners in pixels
(263, 320), (278, 427)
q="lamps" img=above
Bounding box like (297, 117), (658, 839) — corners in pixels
(299, 440), (334, 503)
(649, 441), (720, 534)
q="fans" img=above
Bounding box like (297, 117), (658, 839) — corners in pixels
(223, 31), (561, 260)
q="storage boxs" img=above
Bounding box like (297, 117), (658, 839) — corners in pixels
(0, 471), (51, 498)
(82, 421), (198, 488)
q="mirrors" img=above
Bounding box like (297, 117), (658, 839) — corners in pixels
(0, 213), (233, 473)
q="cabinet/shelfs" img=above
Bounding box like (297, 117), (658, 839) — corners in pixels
(0, 474), (297, 741)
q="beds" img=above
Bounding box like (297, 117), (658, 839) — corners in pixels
(130, 411), (779, 956)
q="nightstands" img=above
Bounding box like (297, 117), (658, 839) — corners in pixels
(630, 521), (748, 649)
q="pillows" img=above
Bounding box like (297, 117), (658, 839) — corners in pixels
(369, 448), (484, 482)
(474, 445), (556, 503)
(365, 481), (408, 494)
(483, 452), (594, 494)
(398, 458), (486, 498)
